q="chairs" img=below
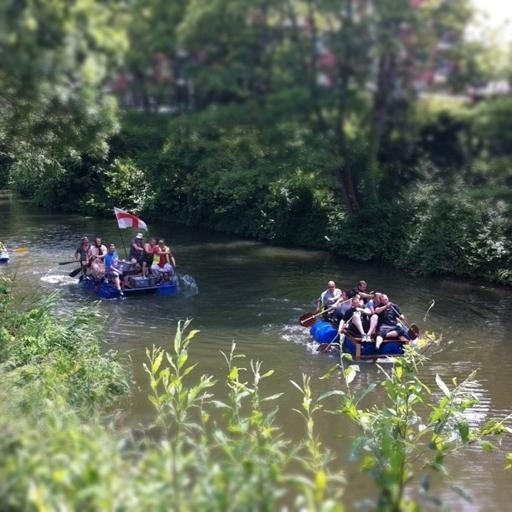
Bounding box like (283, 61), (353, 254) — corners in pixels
(361, 335), (372, 343)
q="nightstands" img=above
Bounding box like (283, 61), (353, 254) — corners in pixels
(316, 316), (353, 352)
(69, 257), (97, 277)
(59, 260), (85, 265)
(300, 298), (352, 327)
(391, 305), (419, 340)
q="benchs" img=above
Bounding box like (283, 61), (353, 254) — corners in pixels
(114, 206), (148, 232)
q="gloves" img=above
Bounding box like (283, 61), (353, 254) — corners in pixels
(0, 242), (9, 261)
(79, 261), (181, 301)
(310, 320), (412, 358)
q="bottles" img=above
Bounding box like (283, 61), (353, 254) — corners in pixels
(136, 233), (143, 239)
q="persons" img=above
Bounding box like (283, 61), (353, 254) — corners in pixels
(316, 279), (420, 352)
(74, 232), (177, 298)
